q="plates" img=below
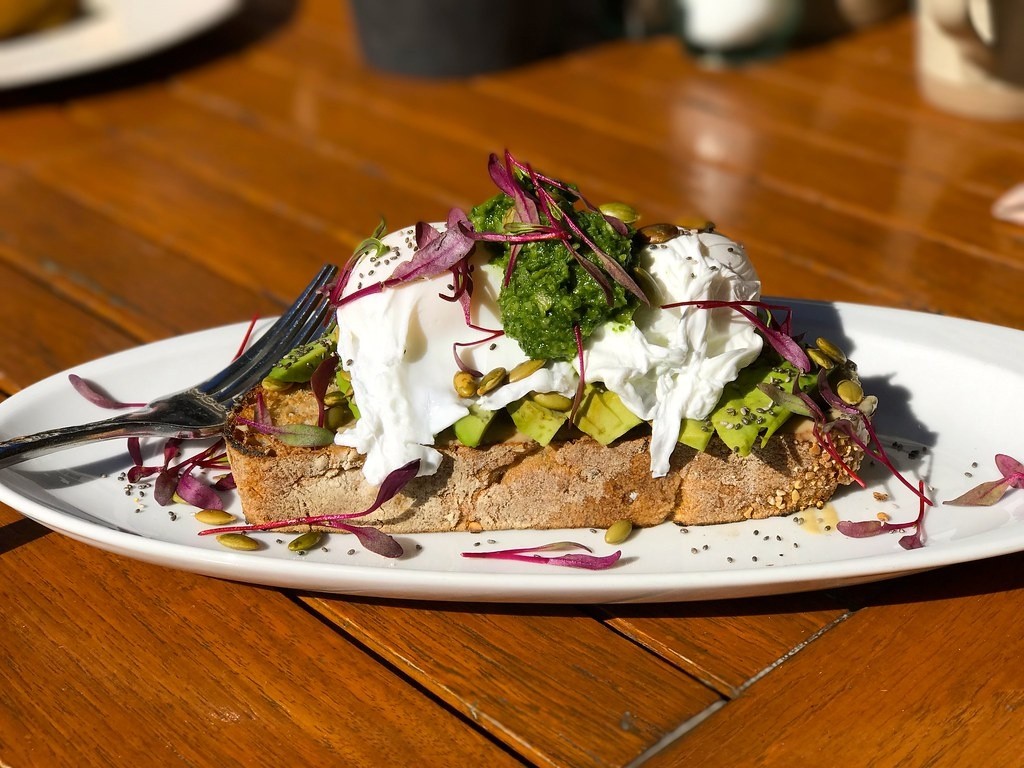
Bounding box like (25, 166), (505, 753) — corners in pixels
(0, 295), (1024, 603)
(0, 0), (242, 89)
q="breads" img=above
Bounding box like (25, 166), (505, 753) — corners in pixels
(224, 356), (871, 531)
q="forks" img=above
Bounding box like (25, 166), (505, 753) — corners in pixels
(0, 265), (337, 471)
